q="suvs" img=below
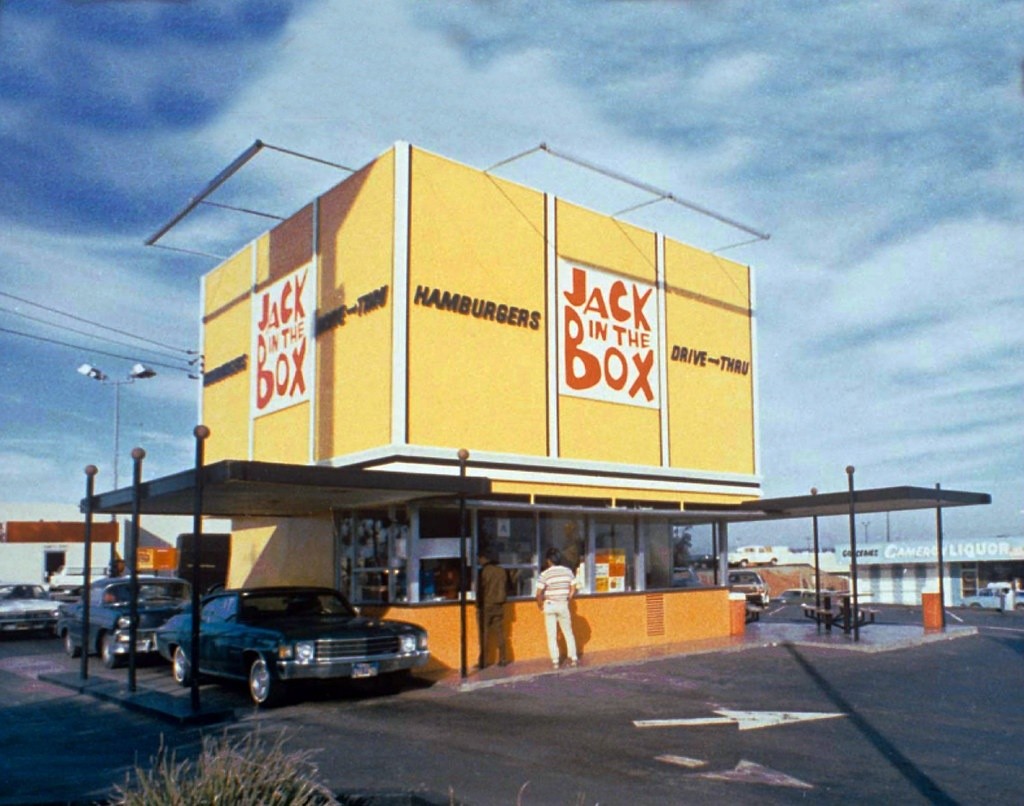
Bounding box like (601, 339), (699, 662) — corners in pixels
(673, 565), (700, 588)
(726, 569), (771, 605)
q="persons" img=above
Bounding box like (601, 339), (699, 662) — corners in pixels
(432, 556), (461, 598)
(535, 549), (581, 666)
(472, 551), (509, 670)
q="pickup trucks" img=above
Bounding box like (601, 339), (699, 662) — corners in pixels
(962, 587), (1024, 611)
(56, 577), (197, 669)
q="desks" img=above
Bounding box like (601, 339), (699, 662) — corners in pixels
(834, 589), (874, 634)
(802, 590), (850, 631)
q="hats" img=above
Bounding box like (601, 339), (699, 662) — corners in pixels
(477, 546), (500, 561)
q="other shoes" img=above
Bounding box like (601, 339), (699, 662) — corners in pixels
(571, 660), (577, 666)
(553, 663), (559, 669)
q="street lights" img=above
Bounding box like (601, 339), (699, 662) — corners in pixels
(76, 360), (156, 571)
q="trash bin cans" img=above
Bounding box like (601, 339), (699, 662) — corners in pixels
(921, 592), (942, 634)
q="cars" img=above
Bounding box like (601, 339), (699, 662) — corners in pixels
(0, 582), (65, 635)
(155, 583), (430, 706)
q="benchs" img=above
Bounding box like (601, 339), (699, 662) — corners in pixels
(835, 600), (881, 629)
(800, 603), (835, 636)
(745, 603), (762, 623)
